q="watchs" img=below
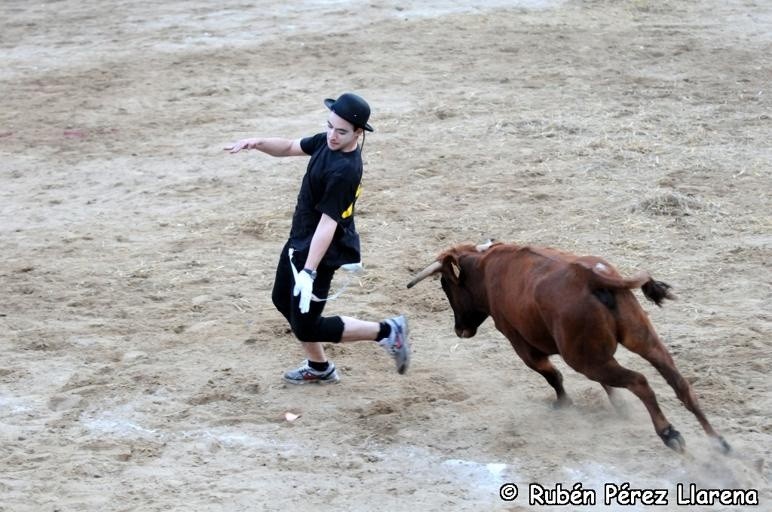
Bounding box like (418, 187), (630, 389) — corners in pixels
(303, 269), (317, 279)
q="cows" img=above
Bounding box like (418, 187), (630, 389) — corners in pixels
(406, 238), (733, 455)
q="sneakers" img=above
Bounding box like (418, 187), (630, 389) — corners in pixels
(376, 314), (410, 375)
(282, 360), (339, 384)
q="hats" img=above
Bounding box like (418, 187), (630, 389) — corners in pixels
(323, 93), (373, 132)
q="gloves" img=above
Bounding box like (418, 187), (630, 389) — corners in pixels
(293, 269), (314, 314)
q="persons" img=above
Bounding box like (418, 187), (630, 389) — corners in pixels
(222, 93), (409, 385)
